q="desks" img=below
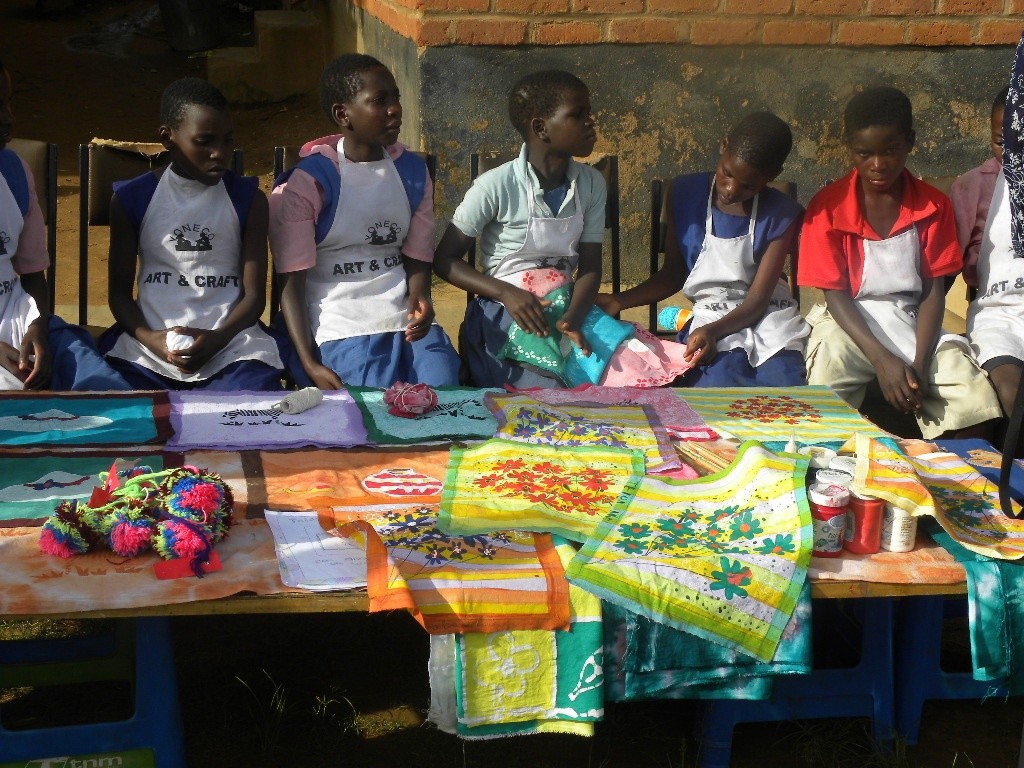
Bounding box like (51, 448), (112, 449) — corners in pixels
(0, 384), (968, 623)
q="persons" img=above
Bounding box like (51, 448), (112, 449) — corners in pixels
(431, 72), (678, 387)
(797, 85), (1024, 459)
(0, 66), (110, 392)
(103, 77), (292, 392)
(591, 110), (808, 386)
(264, 51), (470, 389)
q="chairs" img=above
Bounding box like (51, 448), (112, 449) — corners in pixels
(0, 136), (800, 347)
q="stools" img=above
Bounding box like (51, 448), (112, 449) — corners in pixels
(697, 439), (1024, 768)
(0, 617), (186, 768)
